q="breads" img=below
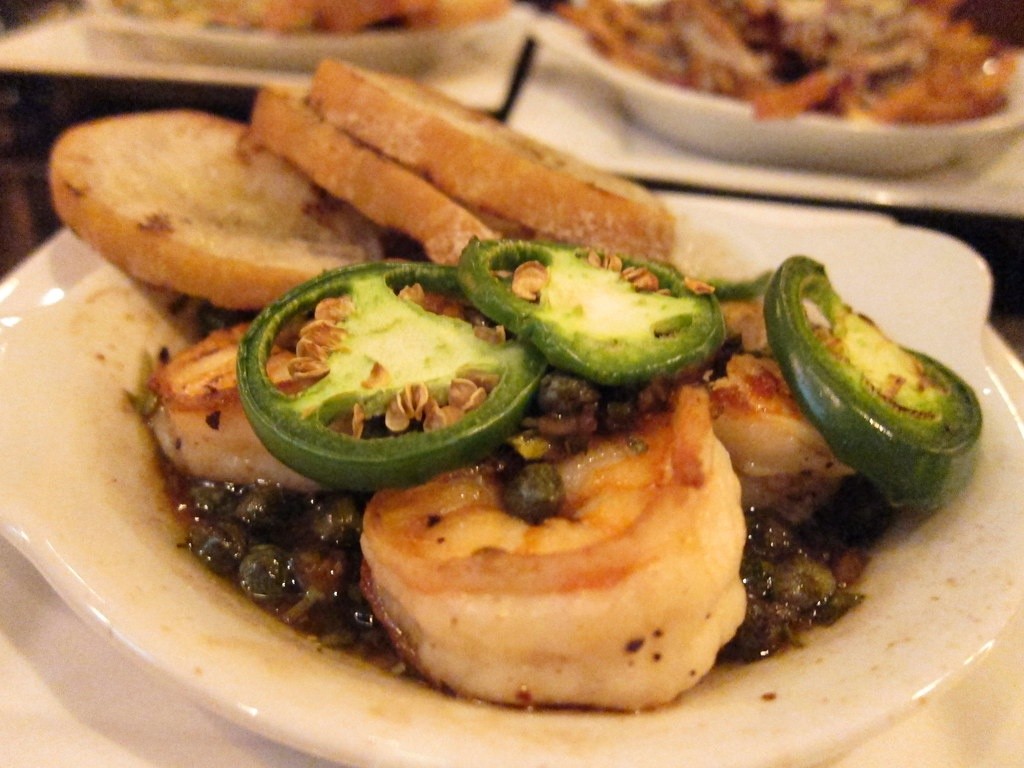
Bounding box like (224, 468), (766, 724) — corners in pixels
(0, 0), (1024, 737)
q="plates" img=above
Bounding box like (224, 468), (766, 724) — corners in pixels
(76, 3), (516, 81)
(531, 6), (1024, 188)
(0, 189), (1023, 767)
